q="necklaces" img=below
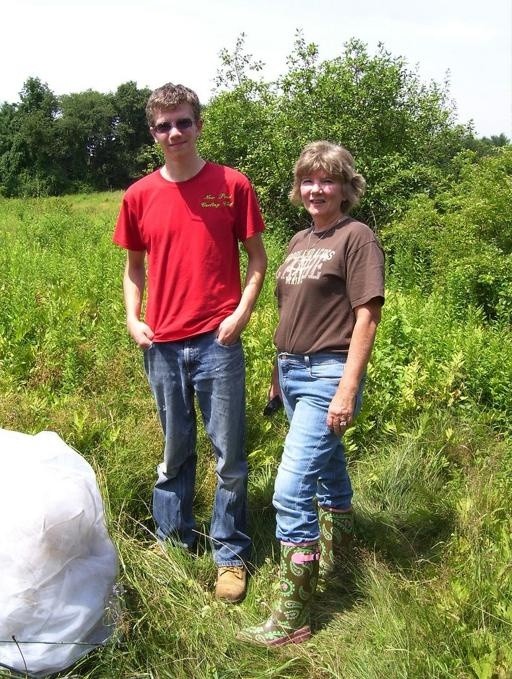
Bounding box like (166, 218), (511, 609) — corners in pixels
(303, 217), (344, 256)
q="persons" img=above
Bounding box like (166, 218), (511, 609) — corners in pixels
(111, 81), (270, 608)
(233, 138), (387, 652)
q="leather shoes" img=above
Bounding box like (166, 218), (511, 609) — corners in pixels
(215, 565), (246, 604)
(146, 542), (164, 557)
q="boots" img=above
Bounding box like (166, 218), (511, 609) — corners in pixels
(235, 507), (355, 650)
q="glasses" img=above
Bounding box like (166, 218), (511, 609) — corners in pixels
(152, 118), (198, 133)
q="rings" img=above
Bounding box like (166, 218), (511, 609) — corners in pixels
(339, 421), (346, 426)
